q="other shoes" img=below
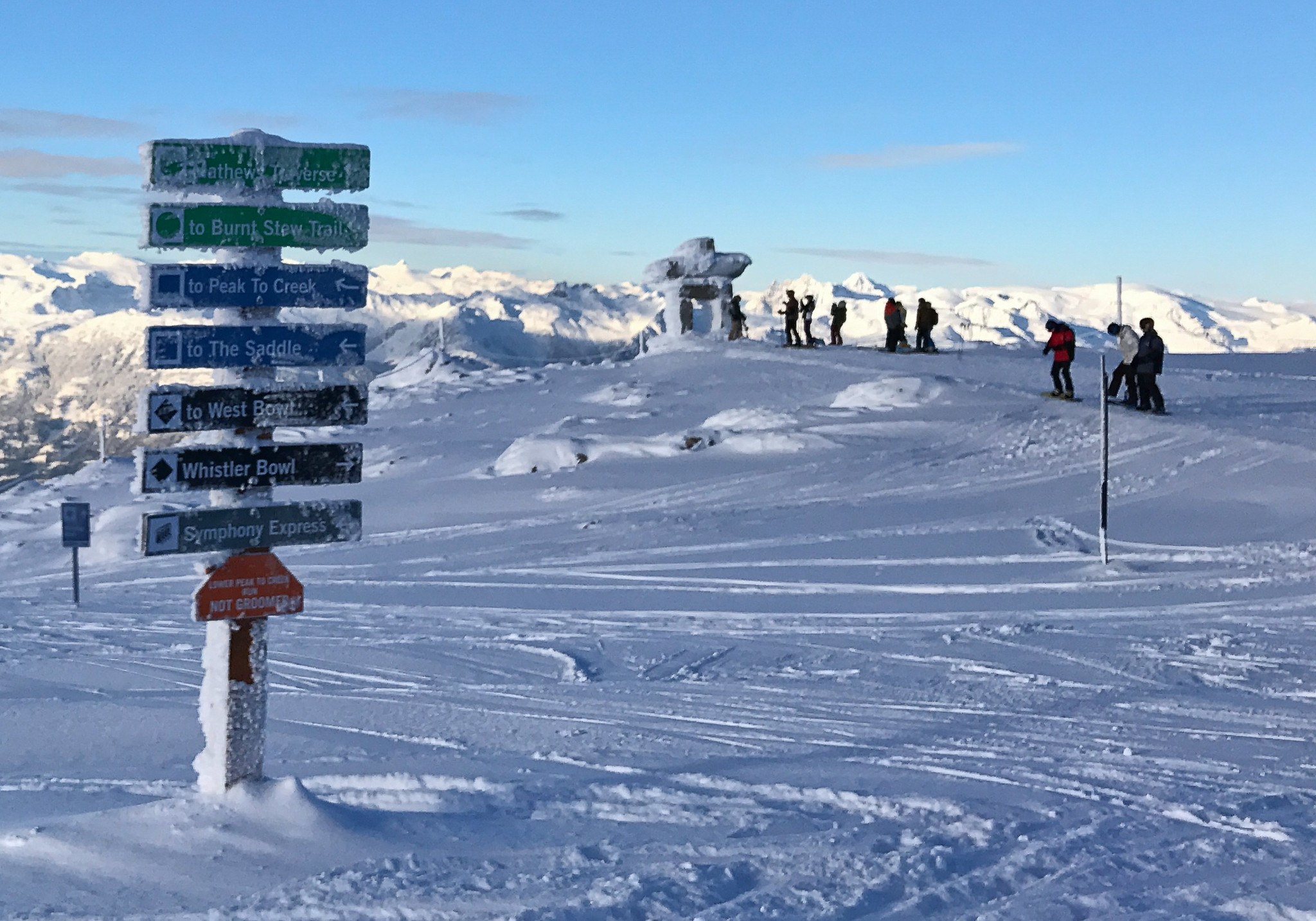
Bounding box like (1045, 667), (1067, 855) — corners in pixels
(1063, 391), (1074, 399)
(1107, 395), (1116, 401)
(836, 342), (843, 346)
(1150, 407), (1166, 413)
(1137, 405), (1151, 411)
(828, 342), (836, 346)
(785, 342), (791, 347)
(1050, 389), (1061, 396)
(794, 342), (801, 346)
(1120, 400), (1137, 406)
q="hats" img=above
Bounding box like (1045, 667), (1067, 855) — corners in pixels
(1045, 320), (1057, 328)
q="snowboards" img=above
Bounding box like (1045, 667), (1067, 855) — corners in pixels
(782, 343), (818, 349)
(826, 344), (852, 347)
(1040, 391), (1082, 403)
(913, 348), (940, 355)
(878, 349), (911, 355)
(1128, 407), (1173, 417)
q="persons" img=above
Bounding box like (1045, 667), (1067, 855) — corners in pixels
(915, 298), (938, 353)
(1106, 322), (1139, 407)
(800, 295), (815, 348)
(884, 297), (905, 352)
(728, 295), (746, 341)
(1042, 319), (1076, 399)
(778, 290), (801, 347)
(895, 301), (912, 349)
(1134, 318), (1165, 414)
(828, 301), (847, 345)
(680, 296), (694, 336)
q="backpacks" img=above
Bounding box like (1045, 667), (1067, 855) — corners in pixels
(923, 306), (939, 326)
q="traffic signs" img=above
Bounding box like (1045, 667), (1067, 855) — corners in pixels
(149, 140), (371, 190)
(144, 500), (363, 558)
(146, 383), (368, 434)
(149, 203), (368, 248)
(149, 263), (368, 309)
(141, 442), (363, 493)
(146, 324), (368, 367)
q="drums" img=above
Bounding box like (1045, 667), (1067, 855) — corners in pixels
(1107, 396), (1139, 409)
